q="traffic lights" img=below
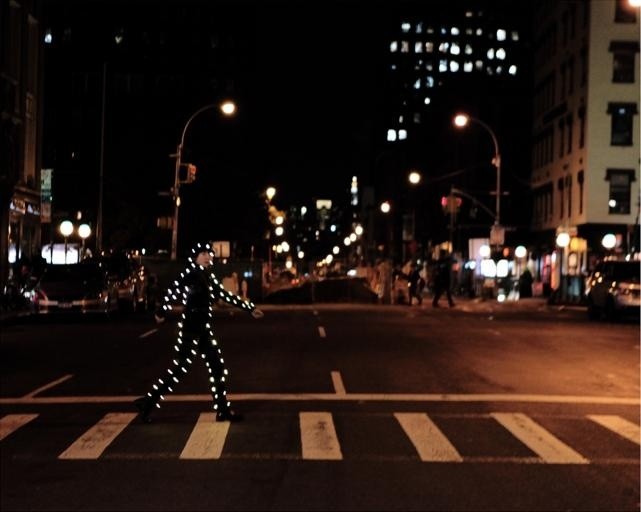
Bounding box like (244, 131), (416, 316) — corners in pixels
(453, 194), (463, 212)
(441, 196), (452, 214)
(179, 160), (197, 185)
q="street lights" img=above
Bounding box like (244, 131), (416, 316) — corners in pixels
(556, 231), (575, 313)
(453, 112), (505, 255)
(265, 172), (424, 288)
(515, 244), (529, 278)
(60, 221), (75, 263)
(171, 98), (236, 259)
(78, 224), (92, 259)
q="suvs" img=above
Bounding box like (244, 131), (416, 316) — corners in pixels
(586, 260), (641, 319)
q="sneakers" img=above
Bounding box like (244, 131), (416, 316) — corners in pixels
(128, 395), (155, 424)
(214, 409), (243, 424)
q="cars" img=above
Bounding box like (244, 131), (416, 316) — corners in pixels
(20, 253), (166, 320)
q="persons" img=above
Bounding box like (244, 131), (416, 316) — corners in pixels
(136, 242), (268, 423)
(405, 263), (423, 305)
(431, 250), (459, 308)
(13, 250), (31, 288)
(31, 248), (47, 284)
